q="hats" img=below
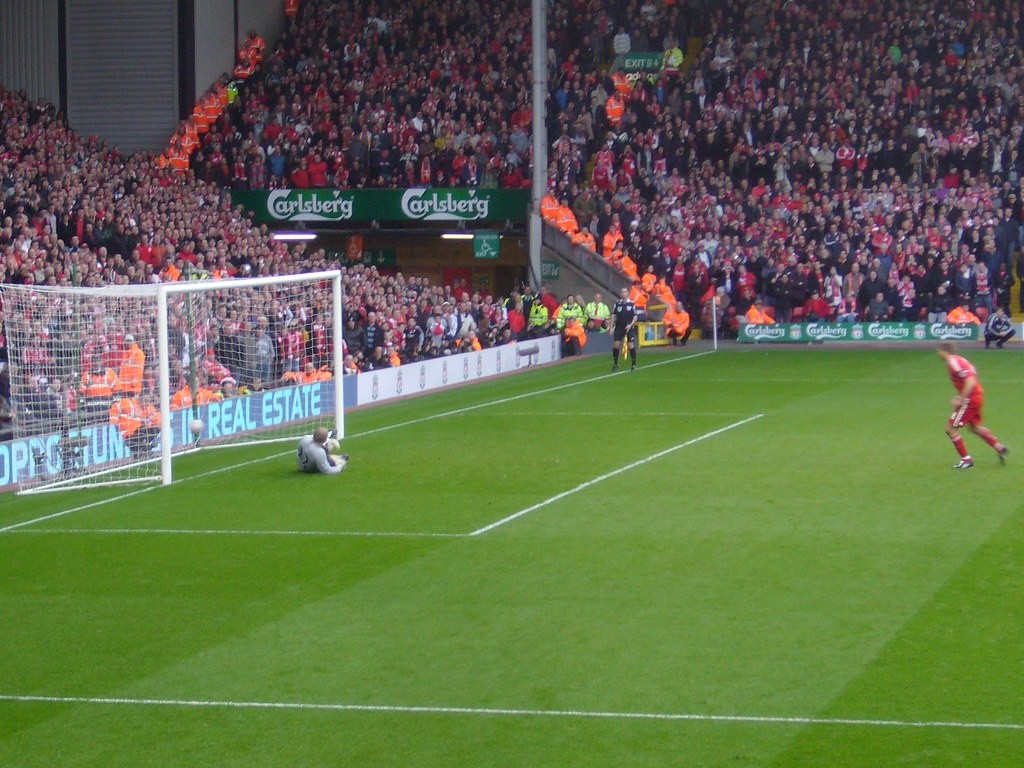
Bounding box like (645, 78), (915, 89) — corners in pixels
(206, 349), (214, 356)
(125, 334), (134, 342)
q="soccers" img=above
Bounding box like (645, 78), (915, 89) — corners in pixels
(190, 419), (204, 433)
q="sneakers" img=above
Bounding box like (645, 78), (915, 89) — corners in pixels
(953, 457), (972, 469)
(999, 446), (1007, 463)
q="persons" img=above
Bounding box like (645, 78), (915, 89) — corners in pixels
(984, 306), (1016, 348)
(0, 83), (519, 461)
(502, 284), (611, 358)
(233, 1), (518, 104)
(610, 287), (639, 372)
(296, 426), (350, 474)
(661, 300), (692, 346)
(510, 0), (1024, 339)
(936, 340), (1007, 468)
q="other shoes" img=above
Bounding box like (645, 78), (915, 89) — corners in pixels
(342, 454), (349, 461)
(631, 364), (637, 373)
(612, 364), (618, 372)
